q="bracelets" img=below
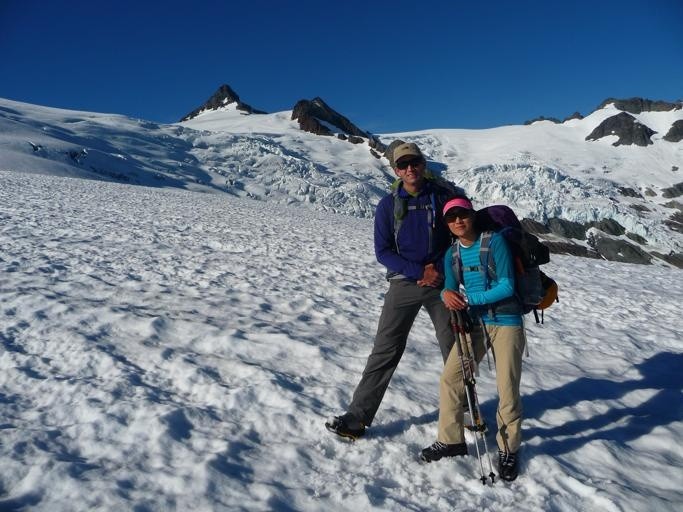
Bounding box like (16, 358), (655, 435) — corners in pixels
(463, 296), (468, 304)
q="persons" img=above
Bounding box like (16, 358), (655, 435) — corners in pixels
(420, 199), (525, 481)
(324, 142), (466, 436)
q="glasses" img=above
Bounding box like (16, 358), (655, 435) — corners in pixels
(397, 158), (424, 170)
(444, 209), (471, 223)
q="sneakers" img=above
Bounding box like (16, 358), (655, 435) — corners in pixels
(498, 451), (518, 481)
(420, 442), (468, 463)
(325, 414), (366, 440)
(463, 408), (486, 431)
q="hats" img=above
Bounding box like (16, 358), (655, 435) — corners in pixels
(441, 196), (474, 218)
(393, 143), (425, 166)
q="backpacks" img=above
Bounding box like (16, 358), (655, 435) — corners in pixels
(473, 205), (558, 314)
